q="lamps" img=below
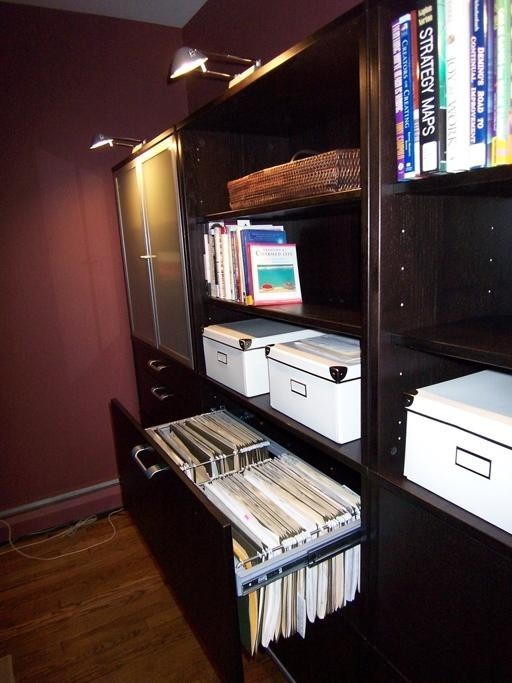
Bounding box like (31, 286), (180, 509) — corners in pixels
(87, 130), (150, 156)
(167, 43), (212, 80)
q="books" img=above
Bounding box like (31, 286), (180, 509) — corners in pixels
(201, 217), (304, 306)
(389, 0), (512, 182)
(147, 409), (366, 658)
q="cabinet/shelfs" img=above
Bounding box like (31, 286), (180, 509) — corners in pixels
(179, 3), (368, 680)
(108, 123), (196, 606)
(361, 2), (511, 682)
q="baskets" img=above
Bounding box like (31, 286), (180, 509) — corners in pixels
(227, 147), (360, 212)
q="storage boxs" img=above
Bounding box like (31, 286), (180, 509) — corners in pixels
(394, 365), (508, 536)
(267, 331), (363, 445)
(199, 307), (318, 402)
